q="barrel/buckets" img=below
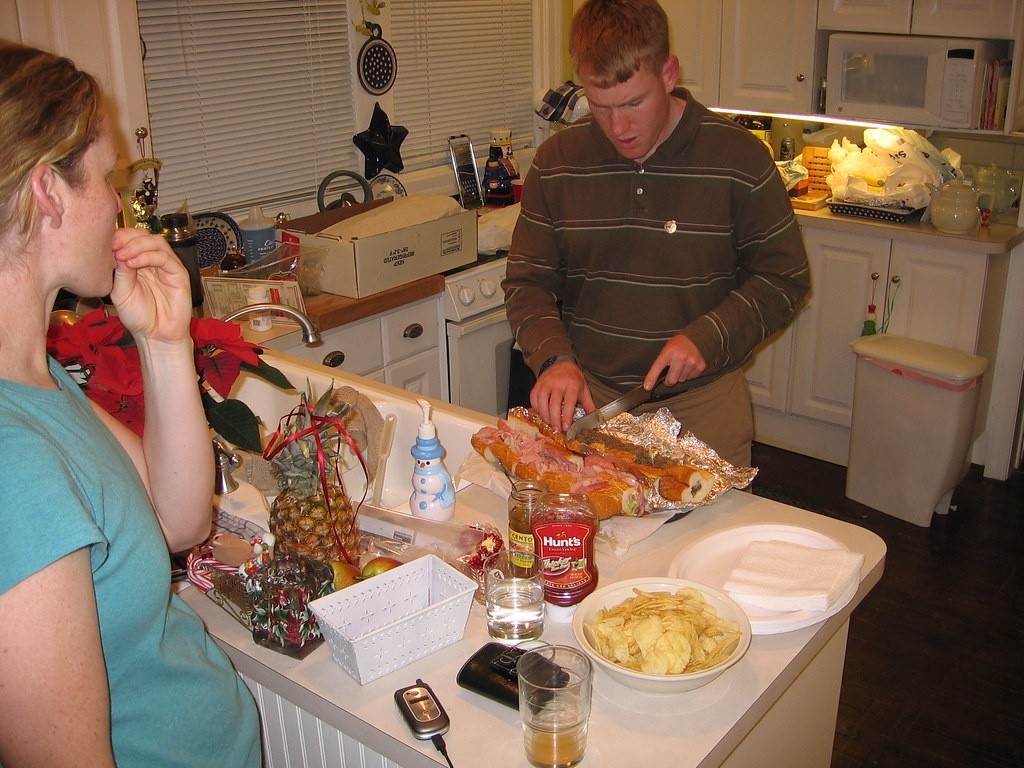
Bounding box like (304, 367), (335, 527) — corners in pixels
(239, 207), (277, 265)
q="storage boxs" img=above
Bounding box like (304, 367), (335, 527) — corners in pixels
(276, 196), (478, 299)
(307, 552), (479, 687)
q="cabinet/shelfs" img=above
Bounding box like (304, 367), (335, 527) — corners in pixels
(656, 0), (1024, 139)
(747, 206), (1024, 465)
(234, 271), (445, 404)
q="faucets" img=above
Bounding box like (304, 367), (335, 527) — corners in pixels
(220, 301), (322, 345)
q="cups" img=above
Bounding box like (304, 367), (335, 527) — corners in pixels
(483, 549), (545, 644)
(510, 179), (525, 204)
(516, 643), (592, 768)
(247, 552), (337, 661)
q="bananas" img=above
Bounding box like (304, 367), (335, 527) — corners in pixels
(854, 174), (888, 192)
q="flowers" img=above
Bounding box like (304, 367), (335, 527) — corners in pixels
(45, 305), (296, 455)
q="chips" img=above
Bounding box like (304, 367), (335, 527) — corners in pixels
(583, 587), (743, 676)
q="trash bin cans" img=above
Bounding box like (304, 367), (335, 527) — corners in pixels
(846, 331), (989, 527)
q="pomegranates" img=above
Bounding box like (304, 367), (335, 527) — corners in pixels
(459, 527), (502, 571)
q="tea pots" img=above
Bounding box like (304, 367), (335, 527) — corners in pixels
(924, 171), (996, 235)
(968, 161), (1022, 215)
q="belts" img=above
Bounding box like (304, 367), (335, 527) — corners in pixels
(592, 378), (687, 405)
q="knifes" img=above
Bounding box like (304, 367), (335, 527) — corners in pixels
(565, 366), (669, 443)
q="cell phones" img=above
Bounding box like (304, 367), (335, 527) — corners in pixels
(394, 679), (450, 740)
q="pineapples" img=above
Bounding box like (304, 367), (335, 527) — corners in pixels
(267, 375), (360, 567)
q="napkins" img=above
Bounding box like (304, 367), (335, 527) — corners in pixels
(723, 540), (866, 614)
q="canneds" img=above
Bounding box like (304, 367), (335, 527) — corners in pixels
(507, 479), (598, 608)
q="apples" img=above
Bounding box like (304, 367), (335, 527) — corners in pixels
(326, 556), (403, 589)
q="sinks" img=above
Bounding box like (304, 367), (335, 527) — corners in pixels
(332, 394), (499, 517)
(207, 355), (355, 448)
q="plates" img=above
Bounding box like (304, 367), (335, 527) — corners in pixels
(669, 523), (861, 636)
(369, 174), (407, 203)
(191, 211), (242, 270)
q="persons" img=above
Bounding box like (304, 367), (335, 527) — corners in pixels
(0, 42), (263, 768)
(502, 0), (812, 494)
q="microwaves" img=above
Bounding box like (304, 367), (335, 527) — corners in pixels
(825, 31), (994, 128)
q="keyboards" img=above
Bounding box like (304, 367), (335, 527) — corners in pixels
(209, 504), (266, 543)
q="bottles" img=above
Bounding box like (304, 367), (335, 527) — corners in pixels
(246, 286), (272, 331)
(507, 480), (548, 576)
(530, 492), (599, 624)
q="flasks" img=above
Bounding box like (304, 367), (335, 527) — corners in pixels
(160, 212), (203, 308)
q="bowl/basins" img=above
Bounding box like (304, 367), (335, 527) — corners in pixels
(572, 577), (752, 696)
